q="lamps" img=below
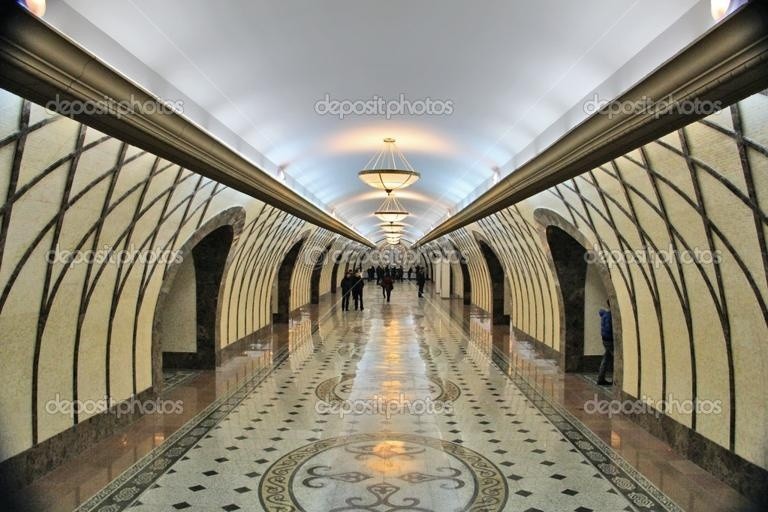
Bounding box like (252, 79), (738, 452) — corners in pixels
(358, 138), (421, 246)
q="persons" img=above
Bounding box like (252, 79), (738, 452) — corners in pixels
(348, 263), (426, 303)
(351, 274), (365, 312)
(339, 272), (350, 312)
(595, 299), (614, 387)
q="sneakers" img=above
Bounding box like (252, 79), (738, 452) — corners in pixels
(597, 378), (613, 386)
